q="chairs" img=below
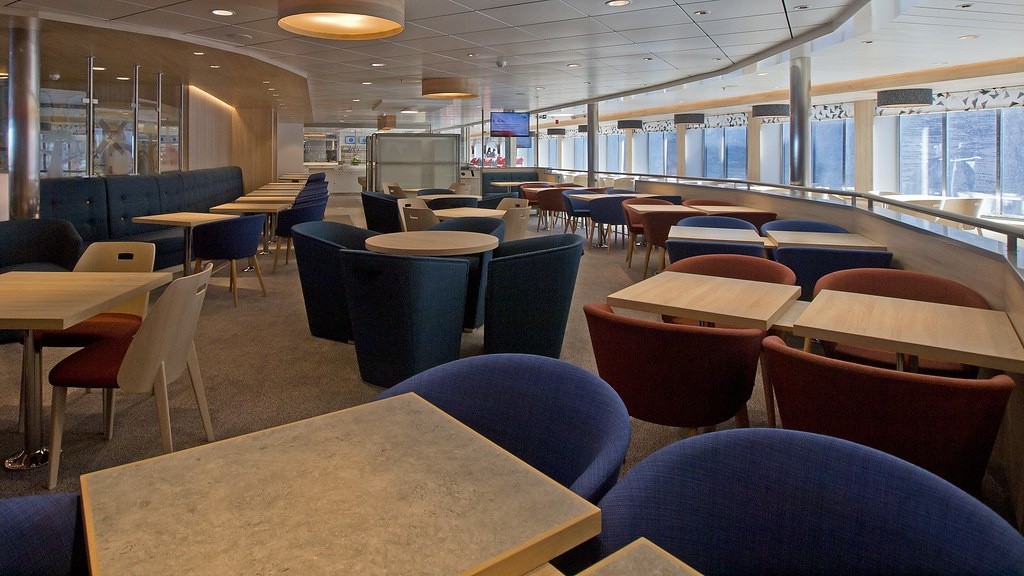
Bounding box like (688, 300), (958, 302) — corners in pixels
(0, 172), (1024, 576)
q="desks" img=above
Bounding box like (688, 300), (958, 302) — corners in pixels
(667, 226), (887, 252)
(416, 193), (482, 202)
(402, 188), (455, 192)
(132, 173), (309, 277)
(569, 193), (662, 253)
(0, 271), (173, 470)
(491, 181), (556, 193)
(366, 231), (499, 256)
(523, 187), (586, 232)
(432, 207), (506, 220)
(79, 393), (705, 576)
(607, 270), (1024, 372)
(628, 205), (768, 279)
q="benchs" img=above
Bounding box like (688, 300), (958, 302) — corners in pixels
(41, 166), (244, 271)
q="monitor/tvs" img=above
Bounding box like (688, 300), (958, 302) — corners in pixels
(490, 112), (530, 137)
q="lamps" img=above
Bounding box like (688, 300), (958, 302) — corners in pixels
(277, 0), (405, 40)
(377, 116), (396, 130)
(547, 129), (565, 135)
(674, 113), (704, 124)
(752, 104), (790, 118)
(877, 89), (932, 108)
(422, 77), (478, 98)
(618, 120), (642, 129)
(578, 125), (587, 132)
